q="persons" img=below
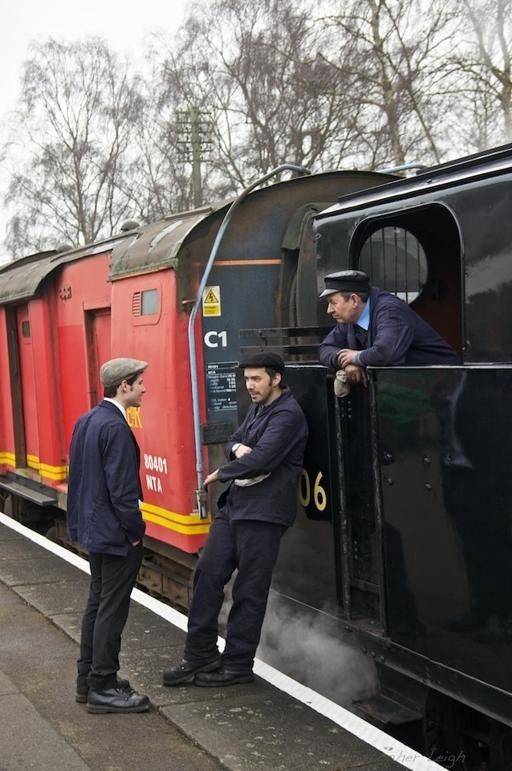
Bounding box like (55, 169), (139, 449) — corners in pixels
(160, 353), (309, 688)
(317, 268), (462, 388)
(66, 358), (154, 715)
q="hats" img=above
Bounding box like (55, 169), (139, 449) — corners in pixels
(100, 357), (152, 389)
(319, 270), (374, 300)
(238, 352), (287, 373)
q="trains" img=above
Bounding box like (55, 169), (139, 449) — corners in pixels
(0, 136), (512, 754)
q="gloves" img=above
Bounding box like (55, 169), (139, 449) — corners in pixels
(333, 365), (352, 398)
(234, 475), (274, 487)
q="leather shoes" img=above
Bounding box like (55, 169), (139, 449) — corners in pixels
(76, 676), (131, 703)
(161, 649), (223, 688)
(195, 659), (257, 686)
(86, 686), (152, 714)
